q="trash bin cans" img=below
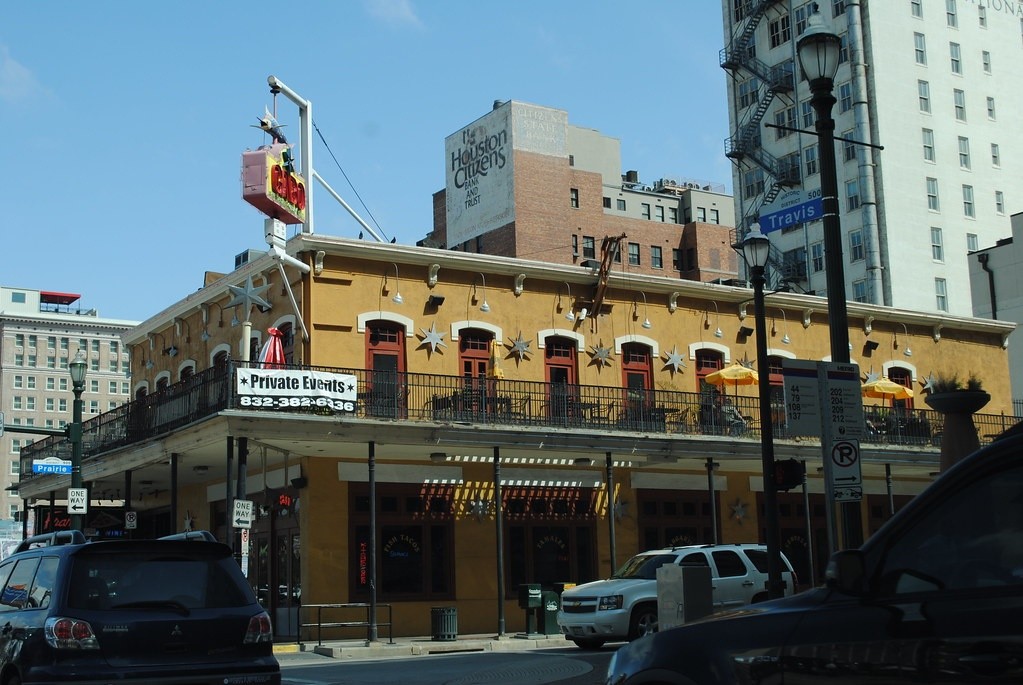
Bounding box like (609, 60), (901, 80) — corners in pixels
(537, 591), (561, 634)
(431, 607), (458, 641)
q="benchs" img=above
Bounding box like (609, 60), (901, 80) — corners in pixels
(980, 440), (990, 448)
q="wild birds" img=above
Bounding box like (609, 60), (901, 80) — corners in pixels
(358, 231), (363, 239)
(391, 237), (396, 243)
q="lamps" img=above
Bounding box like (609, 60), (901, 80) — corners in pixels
(848, 322), (912, 356)
(232, 267), (284, 326)
(706, 300), (789, 345)
(930, 472), (940, 480)
(430, 453), (824, 476)
(126, 332), (166, 378)
(139, 481), (152, 490)
(384, 262), (490, 313)
(559, 283), (651, 330)
(166, 301), (222, 358)
(192, 465), (208, 475)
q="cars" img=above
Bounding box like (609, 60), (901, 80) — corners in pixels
(256, 585), (301, 602)
(606, 421), (1023, 685)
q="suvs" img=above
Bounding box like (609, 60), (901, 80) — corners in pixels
(0, 529), (283, 685)
(556, 543), (801, 649)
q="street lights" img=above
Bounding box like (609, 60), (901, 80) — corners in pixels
(71, 349), (91, 531)
(797, 12), (864, 551)
(742, 222), (785, 599)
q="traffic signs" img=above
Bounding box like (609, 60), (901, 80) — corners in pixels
(231, 500), (252, 529)
(68, 489), (87, 515)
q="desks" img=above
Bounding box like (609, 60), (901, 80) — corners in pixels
(477, 396), (511, 423)
(649, 408), (680, 433)
(983, 434), (1002, 441)
(356, 393), (387, 419)
(560, 403), (598, 428)
(733, 416), (753, 436)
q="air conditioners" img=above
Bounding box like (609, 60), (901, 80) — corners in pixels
(643, 178), (710, 192)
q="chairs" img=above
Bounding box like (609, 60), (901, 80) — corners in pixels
(324, 385), (762, 439)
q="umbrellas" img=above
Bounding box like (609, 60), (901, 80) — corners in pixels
(705, 363), (759, 409)
(860, 376), (914, 418)
(255, 326), (286, 369)
(486, 335), (503, 396)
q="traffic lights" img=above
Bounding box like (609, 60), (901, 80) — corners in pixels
(770, 459), (801, 491)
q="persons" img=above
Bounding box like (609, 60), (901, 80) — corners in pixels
(701, 390), (745, 435)
(865, 403), (881, 423)
